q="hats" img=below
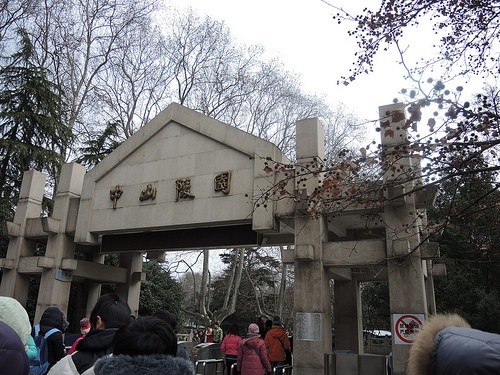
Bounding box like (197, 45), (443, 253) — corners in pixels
(248, 323), (259, 333)
(272, 316), (281, 325)
(80, 318), (92, 334)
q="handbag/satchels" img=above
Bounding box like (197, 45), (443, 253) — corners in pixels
(285, 349), (292, 367)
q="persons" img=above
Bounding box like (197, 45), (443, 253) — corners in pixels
(0, 292), (295, 375)
(408, 313), (500, 375)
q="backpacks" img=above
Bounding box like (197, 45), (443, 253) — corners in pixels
(27, 325), (62, 375)
